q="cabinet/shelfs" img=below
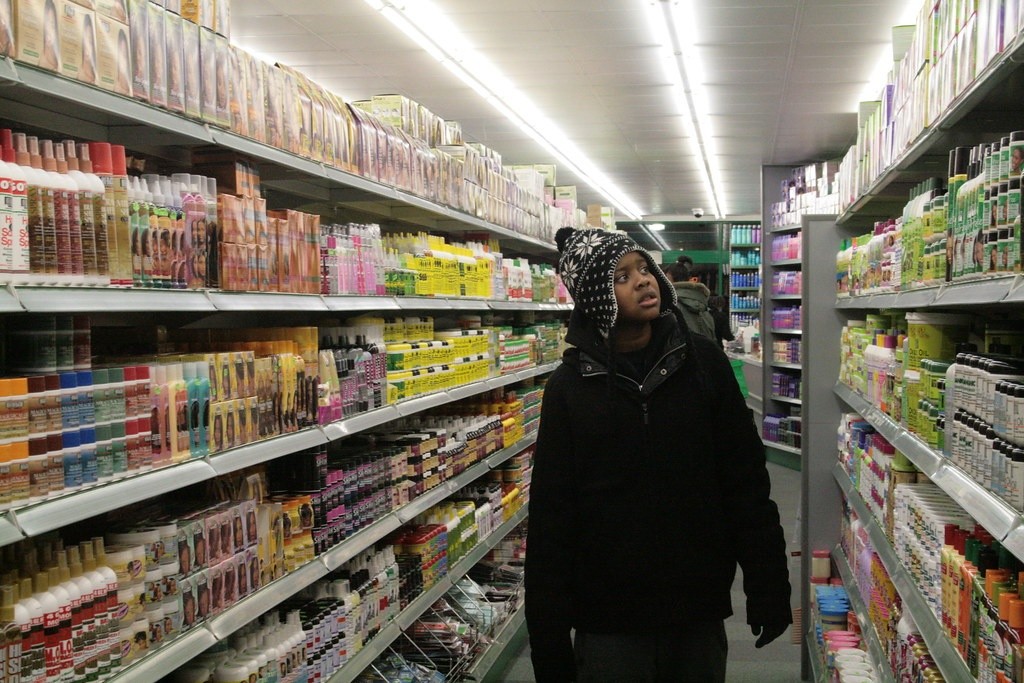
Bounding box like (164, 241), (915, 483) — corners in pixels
(0, 51), (576, 683)
(728, 220), (762, 333)
(804, 28), (1024, 682)
(760, 163), (804, 473)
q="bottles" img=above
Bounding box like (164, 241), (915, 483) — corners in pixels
(731, 293), (761, 309)
(0, 121), (221, 292)
(901, 129), (1024, 290)
(731, 272), (758, 287)
(730, 224), (761, 244)
(731, 249), (760, 267)
(943, 344), (1024, 516)
(732, 313), (760, 336)
(940, 522), (1024, 683)
(0, 530), (119, 683)
(226, 316), (385, 436)
(894, 635), (943, 683)
(835, 218), (895, 300)
(177, 545), (401, 683)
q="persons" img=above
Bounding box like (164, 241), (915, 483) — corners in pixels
(523, 228), (792, 683)
(665, 255), (736, 350)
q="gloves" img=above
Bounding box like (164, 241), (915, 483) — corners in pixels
(534, 666), (577, 683)
(752, 623), (788, 648)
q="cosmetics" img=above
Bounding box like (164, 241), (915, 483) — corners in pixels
(729, 0), (1024, 683)
(0, 1), (613, 683)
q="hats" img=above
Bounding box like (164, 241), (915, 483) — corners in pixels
(555, 228), (710, 433)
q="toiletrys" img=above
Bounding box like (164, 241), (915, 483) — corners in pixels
(835, 131), (1023, 302)
(0, 312), (568, 505)
(836, 309), (1023, 515)
(0, 125), (571, 305)
(0, 453), (530, 683)
(834, 405), (1023, 683)
(729, 223), (761, 333)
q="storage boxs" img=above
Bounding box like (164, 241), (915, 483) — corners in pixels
(0, 0), (615, 633)
(771, 161), (838, 402)
(840, 0), (1024, 218)
